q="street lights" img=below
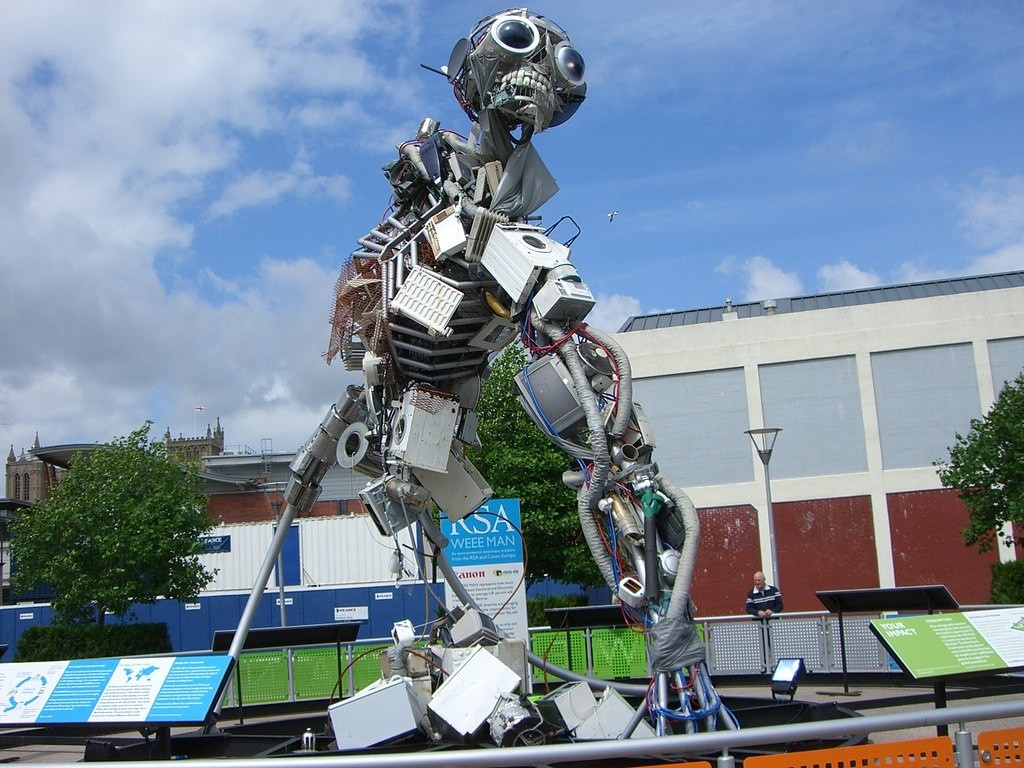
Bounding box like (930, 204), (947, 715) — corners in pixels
(744, 428), (784, 593)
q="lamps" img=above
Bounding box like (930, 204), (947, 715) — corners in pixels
(771, 657), (808, 701)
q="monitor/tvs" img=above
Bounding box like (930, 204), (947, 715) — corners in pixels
(448, 151), (475, 187)
(450, 608), (500, 648)
(468, 317), (516, 351)
(514, 353), (585, 435)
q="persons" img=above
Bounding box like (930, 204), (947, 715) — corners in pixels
(746, 571), (783, 622)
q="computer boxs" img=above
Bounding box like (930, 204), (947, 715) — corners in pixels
(359, 474), (418, 537)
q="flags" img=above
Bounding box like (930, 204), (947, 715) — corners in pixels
(194, 405), (206, 412)
